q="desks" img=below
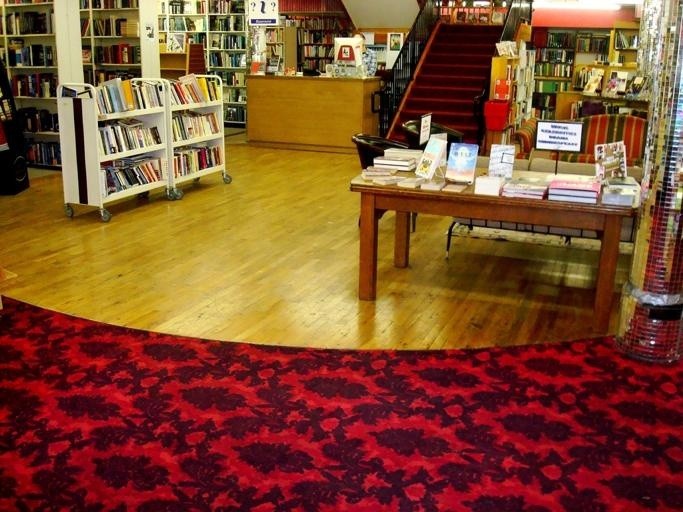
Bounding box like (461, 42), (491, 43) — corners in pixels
(349, 168), (633, 335)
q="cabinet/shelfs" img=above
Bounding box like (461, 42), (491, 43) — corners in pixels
(81, 0), (161, 85)
(248, 0), (353, 75)
(0, 1), (79, 166)
(160, 0), (246, 126)
(53, 74), (231, 221)
(486, 26), (658, 145)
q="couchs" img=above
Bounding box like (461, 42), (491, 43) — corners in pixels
(513, 113), (648, 173)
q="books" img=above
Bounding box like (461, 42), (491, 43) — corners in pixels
(80, 1), (138, 90)
(248, 17), (342, 72)
(158, 2), (247, 122)
(95, 80), (220, 196)
(0, 0), (61, 165)
(359, 148), (637, 207)
(511, 31), (637, 128)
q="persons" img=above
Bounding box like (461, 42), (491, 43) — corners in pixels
(352, 31), (377, 76)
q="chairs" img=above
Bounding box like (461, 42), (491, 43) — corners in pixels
(401, 119), (464, 158)
(351, 133), (417, 232)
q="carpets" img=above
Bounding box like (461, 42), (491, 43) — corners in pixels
(1, 294), (683, 511)
(444, 223), (634, 257)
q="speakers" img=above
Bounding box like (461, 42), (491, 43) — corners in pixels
(0, 146), (29, 195)
